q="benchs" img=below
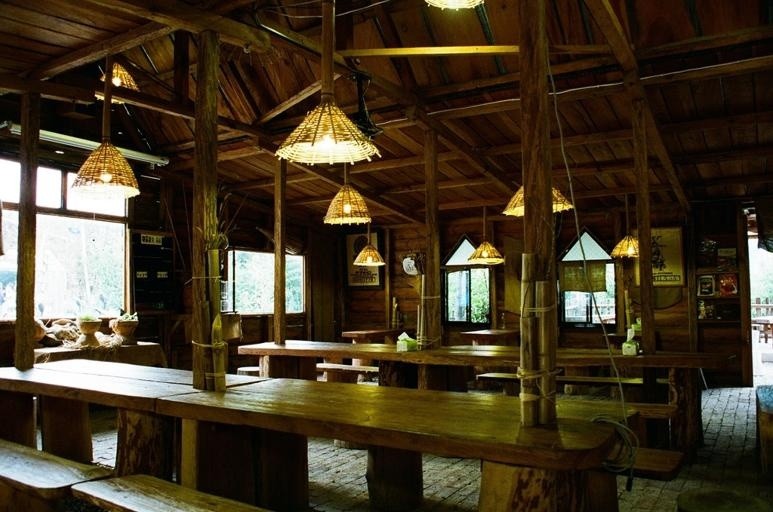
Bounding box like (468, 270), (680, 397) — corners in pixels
(756, 386), (773, 478)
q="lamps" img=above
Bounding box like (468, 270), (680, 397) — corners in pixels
(71, 44), (150, 199)
(275, 1), (382, 165)
(353, 219), (385, 268)
(608, 189), (639, 259)
(322, 164), (374, 226)
(426, 1), (484, 10)
(467, 207), (502, 266)
(95, 61), (139, 108)
(497, 182), (573, 218)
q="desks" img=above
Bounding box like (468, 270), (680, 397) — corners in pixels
(0, 324), (714, 512)
(750, 316), (773, 346)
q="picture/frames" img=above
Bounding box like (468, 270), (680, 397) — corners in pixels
(629, 226), (685, 288)
(344, 229), (384, 291)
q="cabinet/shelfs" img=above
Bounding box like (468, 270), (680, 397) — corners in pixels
(696, 234), (742, 375)
